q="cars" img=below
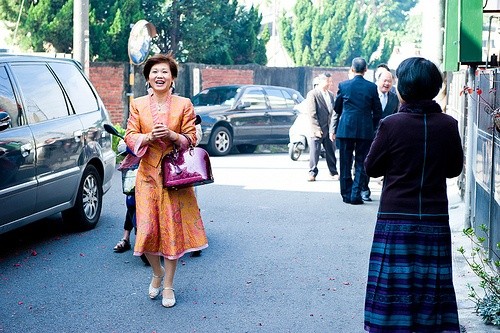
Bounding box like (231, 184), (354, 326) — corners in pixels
(190, 84), (305, 157)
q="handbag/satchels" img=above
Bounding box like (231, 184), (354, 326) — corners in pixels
(163, 133), (214, 190)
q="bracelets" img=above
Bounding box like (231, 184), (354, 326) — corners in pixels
(171, 132), (179, 142)
(146, 132), (151, 143)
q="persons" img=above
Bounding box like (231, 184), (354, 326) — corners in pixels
(328, 57), (401, 205)
(294, 75), (319, 111)
(306, 72), (340, 181)
(110, 80), (202, 258)
(124, 54), (210, 307)
(361, 58), (465, 333)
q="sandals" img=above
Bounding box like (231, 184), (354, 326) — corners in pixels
(148, 265), (165, 298)
(114, 239), (132, 252)
(161, 287), (176, 307)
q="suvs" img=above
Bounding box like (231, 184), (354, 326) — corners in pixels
(0, 53), (116, 231)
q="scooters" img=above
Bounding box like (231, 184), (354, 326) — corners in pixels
(103, 114), (203, 266)
(289, 94), (336, 161)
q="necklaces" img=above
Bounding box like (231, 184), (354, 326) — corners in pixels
(157, 102), (166, 111)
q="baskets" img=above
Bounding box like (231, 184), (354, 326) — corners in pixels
(122, 170), (138, 194)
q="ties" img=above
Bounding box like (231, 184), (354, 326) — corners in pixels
(381, 94), (387, 111)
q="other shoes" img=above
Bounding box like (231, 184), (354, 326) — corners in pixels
(361, 190), (372, 200)
(351, 201), (364, 204)
(307, 172), (316, 181)
(330, 175), (340, 180)
(343, 197), (351, 202)
